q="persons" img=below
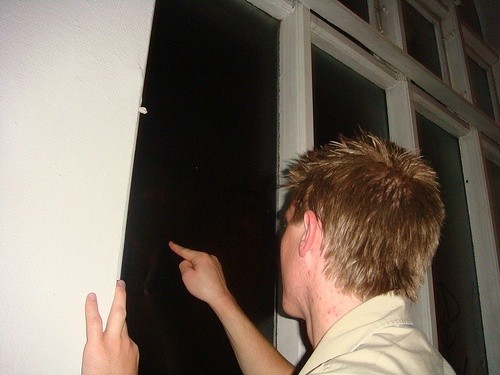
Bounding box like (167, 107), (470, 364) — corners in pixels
(80, 124), (457, 375)
